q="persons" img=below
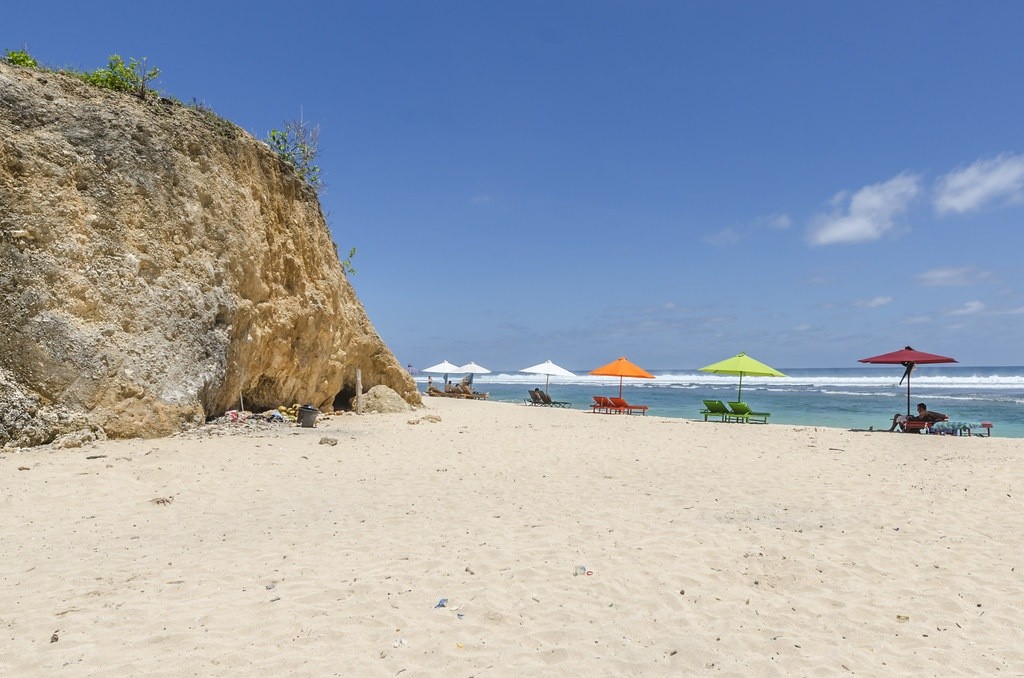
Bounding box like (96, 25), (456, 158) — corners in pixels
(535, 388), (552, 403)
(455, 379), (489, 397)
(425, 376), (437, 397)
(889, 403), (929, 431)
(446, 381), (452, 393)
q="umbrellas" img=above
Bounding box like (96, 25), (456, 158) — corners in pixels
(589, 357), (656, 398)
(460, 362), (491, 389)
(421, 360), (469, 387)
(698, 354), (786, 402)
(857, 346), (959, 415)
(519, 360), (576, 399)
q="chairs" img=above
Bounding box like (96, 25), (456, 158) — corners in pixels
(609, 397), (649, 415)
(700, 400), (739, 422)
(906, 421), (934, 433)
(931, 422), (993, 437)
(538, 391), (572, 409)
(727, 401), (770, 424)
(528, 390), (561, 408)
(427, 386), (490, 401)
(590, 396), (622, 414)
